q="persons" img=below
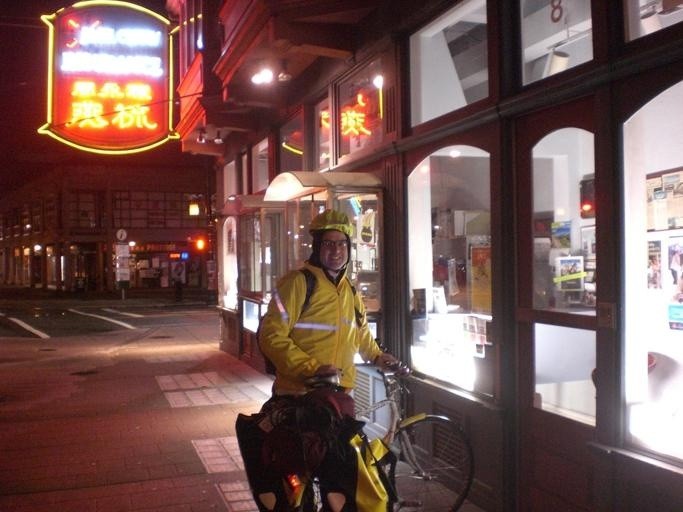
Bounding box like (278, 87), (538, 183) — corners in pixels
(258, 208), (400, 511)
(668, 248), (683, 303)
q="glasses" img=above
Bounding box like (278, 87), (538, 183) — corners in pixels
(320, 239), (349, 248)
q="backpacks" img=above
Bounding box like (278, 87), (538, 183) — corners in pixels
(256, 268), (362, 375)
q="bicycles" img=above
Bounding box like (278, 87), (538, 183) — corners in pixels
(302, 339), (476, 512)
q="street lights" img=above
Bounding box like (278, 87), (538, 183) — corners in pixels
(188, 192), (217, 306)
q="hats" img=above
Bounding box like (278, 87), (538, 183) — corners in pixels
(309, 209), (352, 238)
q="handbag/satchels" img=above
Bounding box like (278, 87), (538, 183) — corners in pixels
(235, 394), (358, 512)
